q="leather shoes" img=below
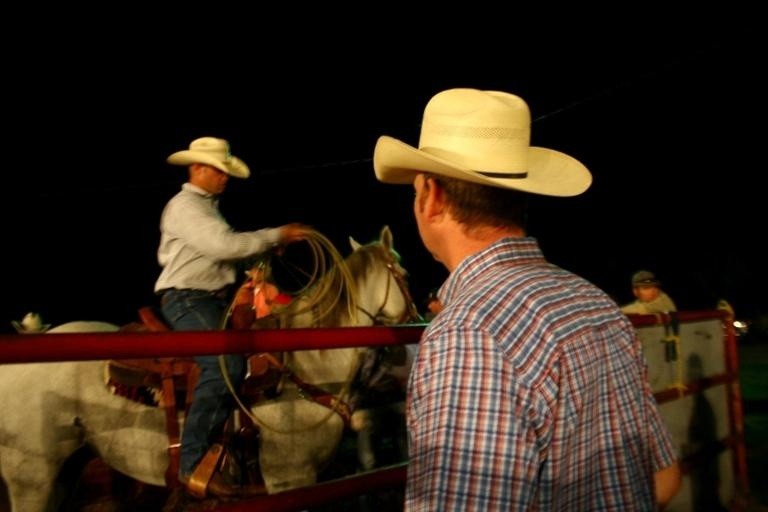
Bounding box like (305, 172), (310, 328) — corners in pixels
(183, 467), (236, 496)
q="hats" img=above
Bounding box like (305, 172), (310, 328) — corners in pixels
(166, 137), (251, 179)
(373, 88), (594, 198)
(426, 289), (439, 302)
(11, 312), (52, 334)
(632, 271), (659, 287)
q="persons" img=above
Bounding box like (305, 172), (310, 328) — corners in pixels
(371, 86), (683, 512)
(618, 270), (677, 316)
(268, 292), (294, 313)
(154, 137), (312, 497)
(232, 261), (279, 321)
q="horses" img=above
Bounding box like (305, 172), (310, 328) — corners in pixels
(1, 224), (423, 512)
